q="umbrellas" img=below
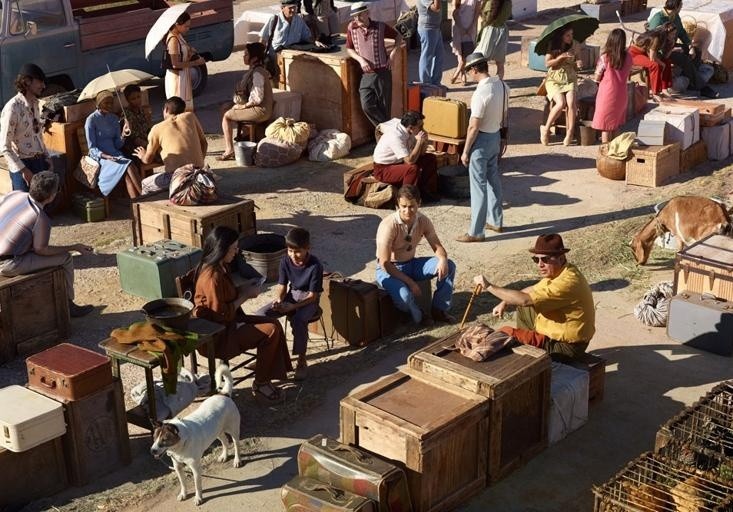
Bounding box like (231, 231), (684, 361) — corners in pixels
(142, 0), (196, 56)
(72, 63), (160, 140)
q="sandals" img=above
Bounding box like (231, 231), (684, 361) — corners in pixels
(250, 379), (286, 403)
(653, 90), (674, 101)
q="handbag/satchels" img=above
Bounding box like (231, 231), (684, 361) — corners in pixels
(161, 35), (184, 71)
(261, 47), (277, 80)
(71, 155), (101, 190)
(461, 41), (474, 52)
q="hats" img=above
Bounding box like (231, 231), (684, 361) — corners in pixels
(281, 0), (299, 9)
(463, 53), (489, 67)
(17, 64), (46, 80)
(528, 233), (571, 255)
(349, 1), (368, 15)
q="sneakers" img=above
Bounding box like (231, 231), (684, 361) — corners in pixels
(540, 124), (575, 149)
(700, 84), (720, 97)
(294, 361), (307, 380)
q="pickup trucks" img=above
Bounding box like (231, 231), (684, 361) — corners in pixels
(0, 0), (234, 111)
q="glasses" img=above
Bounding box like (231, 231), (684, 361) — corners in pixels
(33, 118), (40, 134)
(531, 255), (552, 263)
(404, 235), (414, 251)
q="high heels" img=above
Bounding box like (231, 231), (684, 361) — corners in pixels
(215, 151), (234, 161)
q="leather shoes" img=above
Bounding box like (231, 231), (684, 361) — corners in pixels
(456, 221), (505, 243)
(61, 298), (95, 317)
(402, 306), (458, 329)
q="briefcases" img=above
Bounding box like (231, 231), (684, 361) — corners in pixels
(114, 240), (201, 304)
(672, 97), (727, 128)
(302, 272), (394, 345)
(420, 95), (468, 137)
(1, 341), (112, 455)
(276, 432), (414, 512)
(667, 288), (733, 357)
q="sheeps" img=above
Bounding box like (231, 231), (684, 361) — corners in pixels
(625, 195), (732, 265)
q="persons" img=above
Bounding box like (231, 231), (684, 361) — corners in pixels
(260, 1), (512, 127)
(119, 87), (155, 172)
(0, 61), (55, 220)
(213, 42), (274, 162)
(472, 231), (596, 363)
(83, 90), (146, 201)
(457, 53), (510, 242)
(0, 169), (95, 319)
(541, 1), (720, 147)
(126, 97), (209, 199)
(374, 111), (439, 204)
(252, 224), (325, 386)
(187, 224), (291, 401)
(375, 185), (456, 331)
(162, 13), (205, 122)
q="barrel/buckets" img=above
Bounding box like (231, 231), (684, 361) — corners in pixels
(437, 165), (469, 200)
(233, 140), (256, 168)
(575, 118), (597, 146)
(234, 233), (286, 284)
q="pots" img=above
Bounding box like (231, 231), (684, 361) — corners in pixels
(140, 291), (195, 328)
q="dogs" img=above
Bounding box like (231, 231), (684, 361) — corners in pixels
(149, 365), (244, 506)
